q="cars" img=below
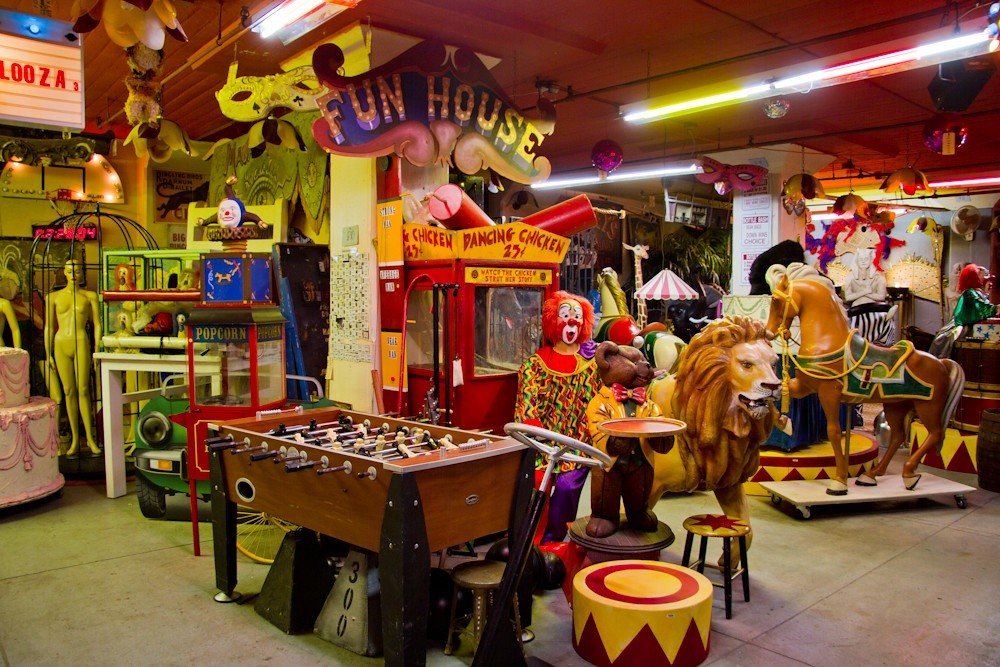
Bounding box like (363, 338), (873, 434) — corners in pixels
(134, 371), (338, 519)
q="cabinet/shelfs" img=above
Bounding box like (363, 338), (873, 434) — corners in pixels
(187, 199), (290, 256)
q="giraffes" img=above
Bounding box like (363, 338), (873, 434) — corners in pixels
(623, 241), (649, 332)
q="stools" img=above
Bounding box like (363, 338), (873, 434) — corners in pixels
(573, 562), (713, 667)
(681, 512), (752, 620)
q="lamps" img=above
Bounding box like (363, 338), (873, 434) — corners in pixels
(878, 127), (934, 192)
(616, 1), (1000, 125)
(530, 122), (704, 189)
(781, 138), (827, 206)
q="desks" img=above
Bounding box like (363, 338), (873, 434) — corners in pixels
(204, 408), (537, 667)
(91, 349), (283, 504)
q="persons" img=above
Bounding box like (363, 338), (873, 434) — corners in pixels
(512, 289), (600, 544)
(585, 340), (674, 537)
(43, 259), (102, 455)
(0, 298), (21, 349)
(843, 248), (887, 307)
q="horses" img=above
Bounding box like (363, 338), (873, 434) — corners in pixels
(595, 268), (687, 374)
(763, 273), (966, 496)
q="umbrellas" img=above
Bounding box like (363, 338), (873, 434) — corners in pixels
(634, 269), (700, 301)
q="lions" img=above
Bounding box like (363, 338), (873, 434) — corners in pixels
(648, 316), (784, 574)
